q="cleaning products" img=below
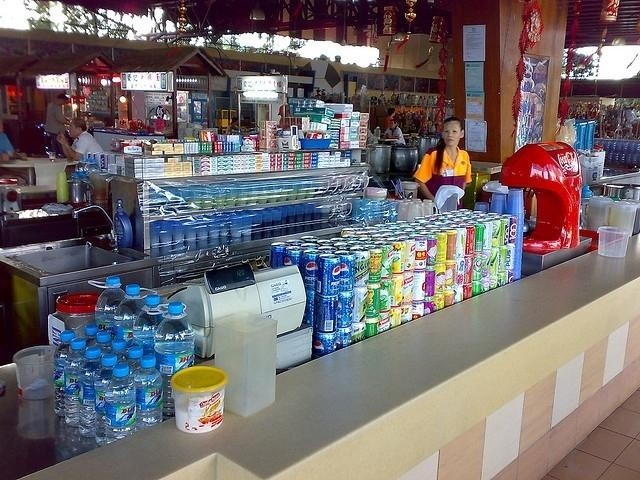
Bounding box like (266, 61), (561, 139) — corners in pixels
(55, 169), (70, 204)
(113, 199), (134, 249)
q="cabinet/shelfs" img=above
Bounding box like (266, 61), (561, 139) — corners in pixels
(110, 163), (373, 252)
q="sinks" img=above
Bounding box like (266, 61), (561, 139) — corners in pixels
(0, 237), (163, 286)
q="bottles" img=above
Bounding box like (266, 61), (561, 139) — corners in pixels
(374, 126), (381, 140)
(0, 179), (22, 212)
(121, 73), (166, 89)
(309, 87), (361, 106)
(80, 112), (94, 131)
(376, 87), (439, 105)
(403, 132), (441, 147)
(56, 171), (69, 203)
(46, 275), (197, 445)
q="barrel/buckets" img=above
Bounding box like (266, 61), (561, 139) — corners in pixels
(592, 150), (606, 178)
(366, 132), (442, 178)
(596, 226), (630, 258)
(16, 400), (58, 439)
(366, 186), (388, 200)
(588, 182), (640, 236)
(70, 176), (95, 208)
(397, 181), (438, 222)
(581, 156), (600, 186)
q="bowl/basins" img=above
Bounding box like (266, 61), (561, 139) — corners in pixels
(298, 138), (332, 149)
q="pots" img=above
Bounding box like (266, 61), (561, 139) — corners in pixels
(392, 146), (418, 173)
(365, 144), (391, 173)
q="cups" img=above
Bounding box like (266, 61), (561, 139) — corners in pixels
(18, 398), (53, 439)
(474, 188), (524, 283)
(578, 138), (640, 257)
(72, 176), (93, 203)
(49, 151), (56, 160)
(351, 186), (438, 228)
(149, 202), (329, 247)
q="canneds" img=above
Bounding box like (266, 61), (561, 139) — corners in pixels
(270, 208), (518, 360)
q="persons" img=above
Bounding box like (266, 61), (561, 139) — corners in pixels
(383, 109), (397, 129)
(44, 94), (70, 157)
(0, 121), (17, 163)
(412, 118), (473, 211)
(56, 119), (104, 161)
(382, 121), (407, 146)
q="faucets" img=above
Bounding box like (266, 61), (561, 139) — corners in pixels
(72, 204), (118, 252)
